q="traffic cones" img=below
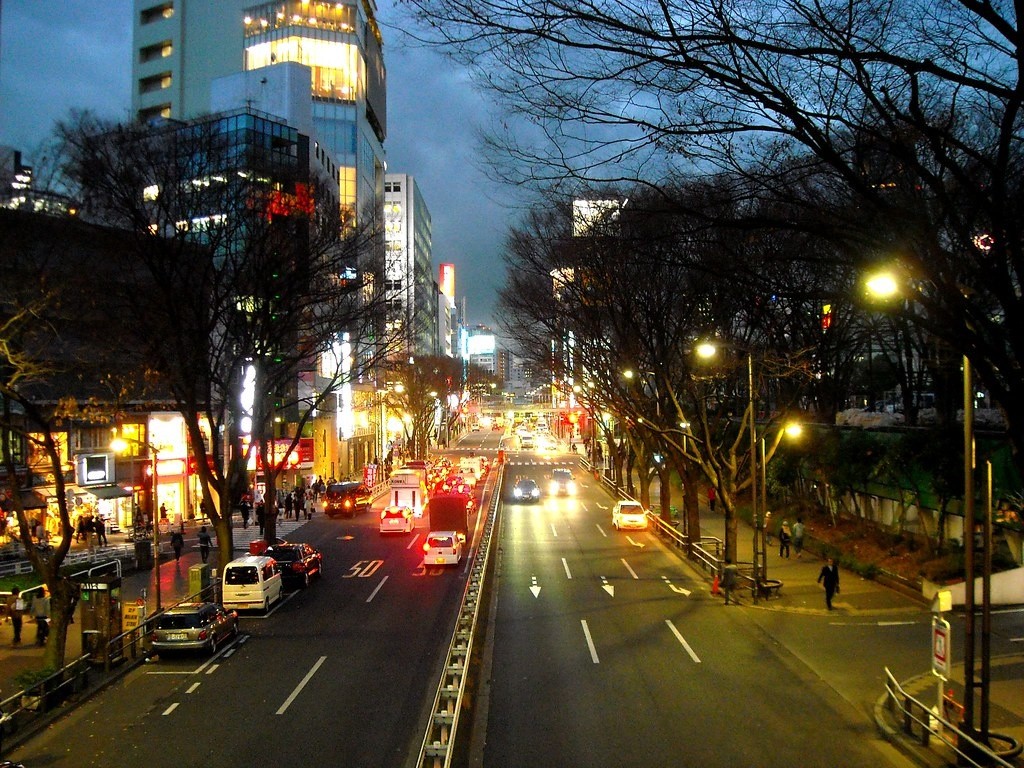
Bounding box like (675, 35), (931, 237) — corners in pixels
(709, 574), (721, 595)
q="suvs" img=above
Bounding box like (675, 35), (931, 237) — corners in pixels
(322, 480), (374, 519)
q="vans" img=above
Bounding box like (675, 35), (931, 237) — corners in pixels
(221, 555), (284, 615)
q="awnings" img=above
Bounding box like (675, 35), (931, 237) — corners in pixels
(0, 479), (88, 513)
(87, 483), (133, 501)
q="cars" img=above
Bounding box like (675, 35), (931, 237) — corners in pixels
(422, 530), (463, 569)
(550, 468), (574, 496)
(514, 425), (557, 450)
(151, 602), (240, 657)
(379, 505), (415, 534)
(264, 541), (324, 590)
(383, 453), (490, 546)
(612, 500), (650, 531)
(511, 474), (541, 503)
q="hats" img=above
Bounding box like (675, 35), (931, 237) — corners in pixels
(766, 511), (772, 518)
(783, 521), (789, 526)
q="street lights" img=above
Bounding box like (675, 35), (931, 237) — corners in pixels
(110, 436), (163, 611)
(361, 383), (404, 483)
(697, 340), (802, 597)
(864, 266), (978, 768)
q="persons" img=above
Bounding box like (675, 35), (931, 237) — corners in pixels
(719, 557), (744, 606)
(791, 516), (806, 559)
(751, 564), (769, 597)
(707, 483), (723, 514)
(196, 526), (214, 564)
(200, 499), (211, 522)
(779, 521), (792, 560)
(159, 503), (169, 519)
(273, 474), (356, 528)
(186, 503), (195, 520)
(134, 503), (142, 527)
(5, 586), (24, 645)
(763, 510), (774, 545)
(817, 557), (840, 610)
(30, 588), (51, 647)
(76, 513), (112, 548)
(240, 501), (266, 536)
(169, 528), (185, 562)
(374, 435), (416, 482)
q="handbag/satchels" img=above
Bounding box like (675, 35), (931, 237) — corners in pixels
(836, 586), (840, 593)
